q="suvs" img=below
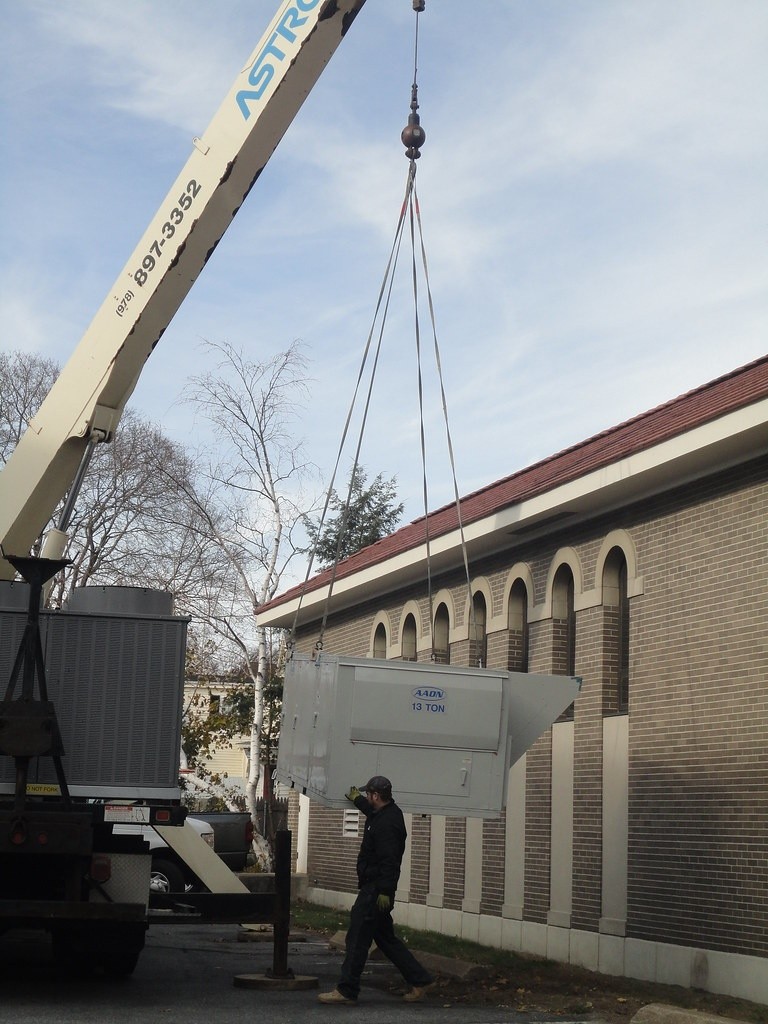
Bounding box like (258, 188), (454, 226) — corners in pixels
(112, 818), (217, 896)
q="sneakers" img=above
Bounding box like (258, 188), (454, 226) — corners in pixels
(318, 990), (357, 1006)
(402, 981), (437, 1002)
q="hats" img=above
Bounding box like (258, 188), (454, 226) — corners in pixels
(358, 776), (392, 795)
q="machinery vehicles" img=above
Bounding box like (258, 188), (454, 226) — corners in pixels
(0, 0), (585, 987)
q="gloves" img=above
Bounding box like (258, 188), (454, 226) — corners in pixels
(344, 785), (361, 801)
(376, 893), (391, 914)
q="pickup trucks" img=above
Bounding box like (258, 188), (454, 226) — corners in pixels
(182, 809), (254, 892)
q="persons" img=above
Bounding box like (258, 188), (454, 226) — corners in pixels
(318, 775), (437, 1002)
